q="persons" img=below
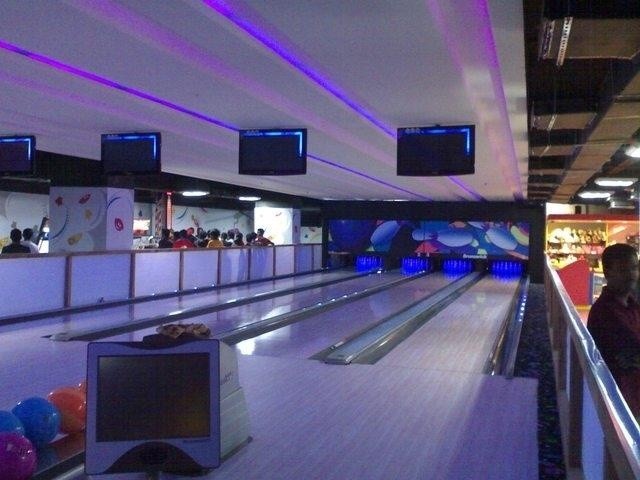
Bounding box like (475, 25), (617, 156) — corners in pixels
(586, 243), (640, 427)
(19, 227), (41, 254)
(158, 227), (275, 250)
(2, 228), (31, 255)
(546, 225), (606, 269)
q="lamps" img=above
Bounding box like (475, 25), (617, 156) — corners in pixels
(239, 196), (261, 201)
(622, 143), (640, 158)
(178, 190), (210, 197)
(578, 190), (615, 199)
(594, 177), (639, 187)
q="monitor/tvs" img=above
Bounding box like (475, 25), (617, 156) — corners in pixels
(85, 339), (250, 475)
(397, 125), (476, 176)
(100, 131), (161, 176)
(0, 135), (36, 178)
(239, 128), (307, 176)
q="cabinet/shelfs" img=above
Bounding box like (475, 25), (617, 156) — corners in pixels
(546, 214), (608, 273)
